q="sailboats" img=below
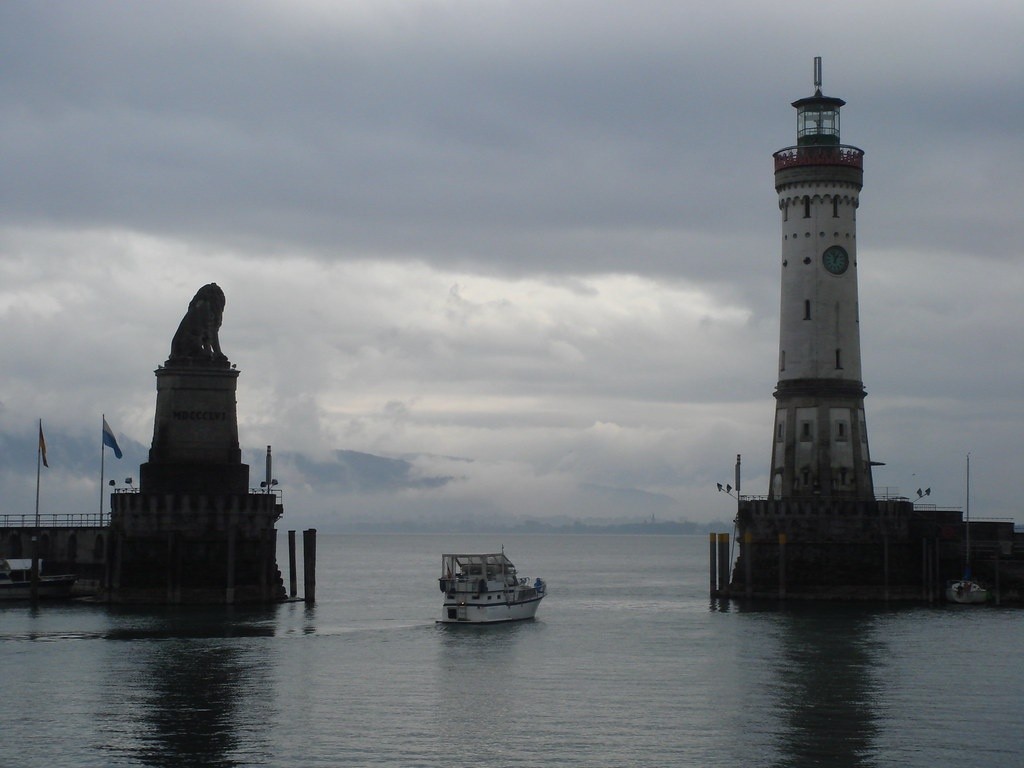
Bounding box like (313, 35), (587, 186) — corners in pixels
(944, 454), (988, 604)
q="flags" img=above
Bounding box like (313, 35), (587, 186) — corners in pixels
(103, 419), (123, 458)
(39, 422), (49, 467)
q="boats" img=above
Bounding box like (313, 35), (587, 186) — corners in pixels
(435, 543), (549, 625)
(0, 558), (80, 601)
